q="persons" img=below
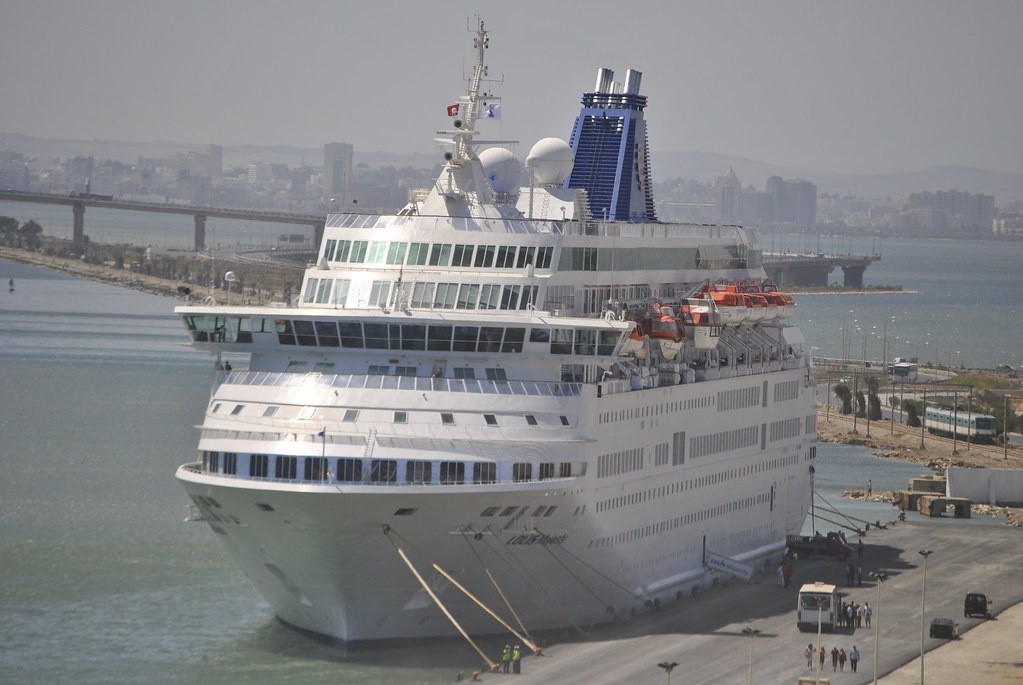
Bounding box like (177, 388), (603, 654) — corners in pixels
(774, 478), (873, 674)
(512, 645), (521, 674)
(502, 644), (511, 674)
(224, 361), (232, 370)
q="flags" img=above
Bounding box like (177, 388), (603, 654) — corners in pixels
(482, 102), (501, 119)
(447, 104), (459, 116)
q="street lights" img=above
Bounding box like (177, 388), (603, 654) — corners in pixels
(918, 549), (933, 685)
(869, 570), (888, 684)
(801, 307), (961, 388)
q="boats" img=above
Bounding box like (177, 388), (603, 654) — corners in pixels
(597, 277), (797, 359)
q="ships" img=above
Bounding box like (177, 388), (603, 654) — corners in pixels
(170, 20), (817, 641)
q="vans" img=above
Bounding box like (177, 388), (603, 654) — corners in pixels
(798, 581), (841, 633)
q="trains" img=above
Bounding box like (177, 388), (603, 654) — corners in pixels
(925, 404), (997, 444)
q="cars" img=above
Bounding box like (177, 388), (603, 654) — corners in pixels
(964, 592), (993, 618)
(6, 179), (118, 208)
(930, 618), (959, 640)
